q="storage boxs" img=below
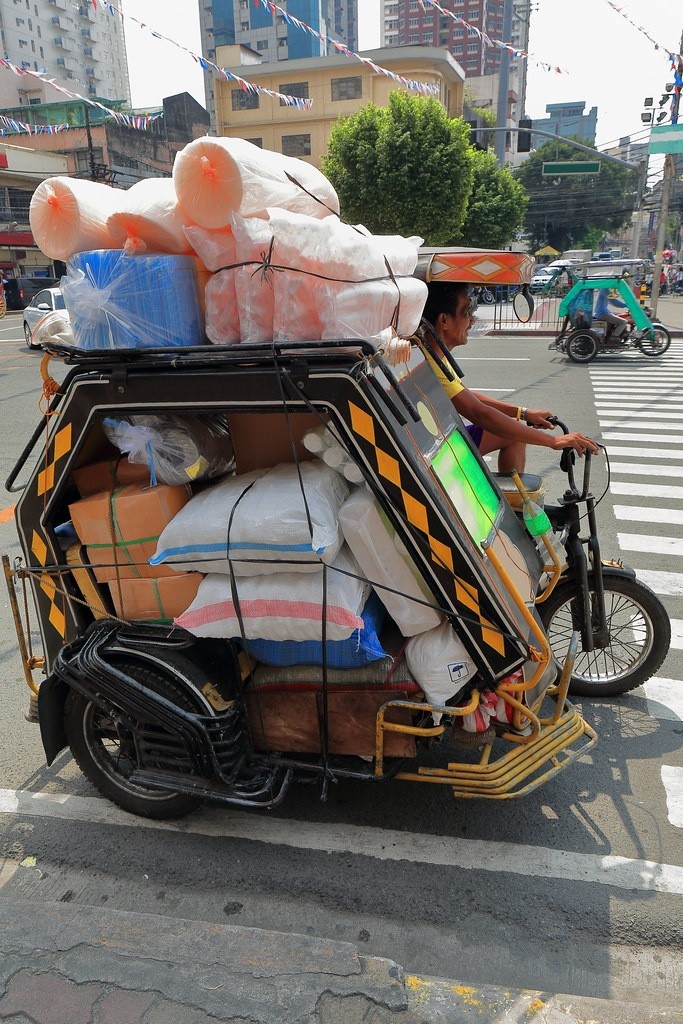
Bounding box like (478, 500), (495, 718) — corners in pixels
(68, 454), (203, 621)
(226, 412), (330, 475)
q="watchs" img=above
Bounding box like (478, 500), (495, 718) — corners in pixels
(520, 407), (527, 420)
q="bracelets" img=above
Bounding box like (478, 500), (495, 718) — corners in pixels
(516, 406), (522, 421)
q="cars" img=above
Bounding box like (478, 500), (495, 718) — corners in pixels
(529, 267), (559, 295)
(23, 288), (66, 350)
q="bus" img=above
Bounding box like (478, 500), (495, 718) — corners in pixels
(599, 250), (621, 261)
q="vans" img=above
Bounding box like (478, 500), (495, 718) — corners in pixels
(5, 276), (60, 311)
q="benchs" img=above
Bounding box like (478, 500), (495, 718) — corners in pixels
(590, 320), (608, 336)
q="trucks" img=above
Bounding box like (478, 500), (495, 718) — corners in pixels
(549, 248), (599, 282)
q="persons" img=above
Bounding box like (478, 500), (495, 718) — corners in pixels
(557, 267), (574, 296)
(594, 278), (629, 336)
(622, 265), (683, 290)
(407, 281), (599, 476)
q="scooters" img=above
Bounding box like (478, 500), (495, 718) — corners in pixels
(476, 286), (495, 304)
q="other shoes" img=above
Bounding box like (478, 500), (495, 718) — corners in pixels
(606, 340), (618, 347)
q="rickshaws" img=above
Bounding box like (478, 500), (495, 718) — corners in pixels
(548, 274), (671, 363)
(645, 263), (683, 298)
(1, 246), (673, 825)
